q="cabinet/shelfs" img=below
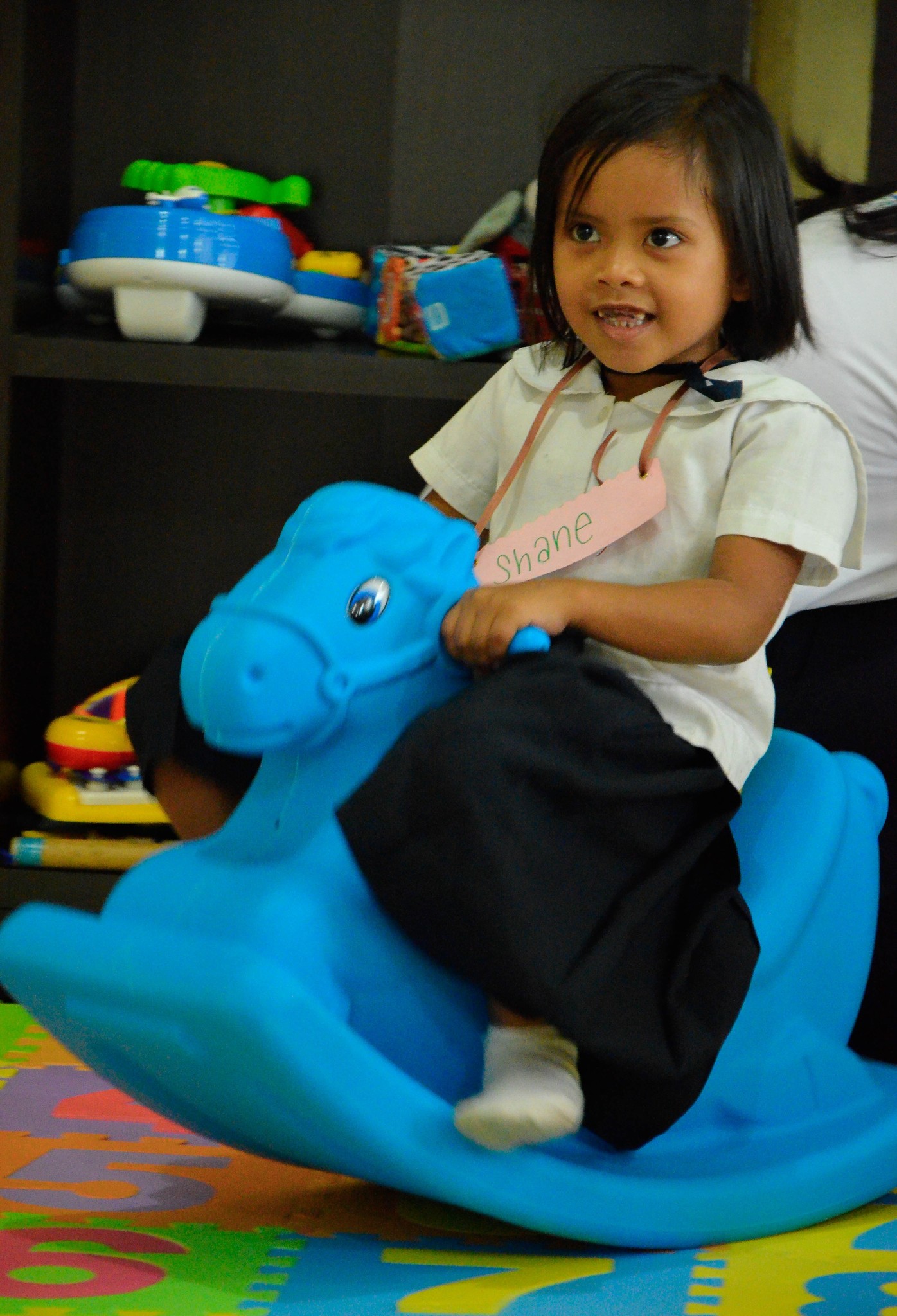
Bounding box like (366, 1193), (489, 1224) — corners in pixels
(1, 1), (748, 1005)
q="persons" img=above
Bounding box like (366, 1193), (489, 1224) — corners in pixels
(334, 62), (865, 1154)
(761, 132), (897, 1064)
